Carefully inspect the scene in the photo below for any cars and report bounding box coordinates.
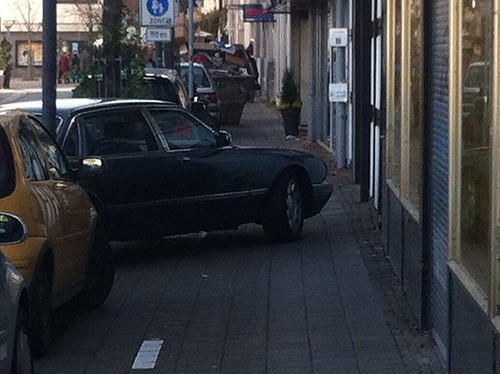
[0,214,36,372]
[0,110,117,348]
[165,62,223,125]
[1,99,333,241]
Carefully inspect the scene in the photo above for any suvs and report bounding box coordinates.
[71,67,208,122]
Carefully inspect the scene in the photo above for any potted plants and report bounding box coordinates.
[280,68,302,138]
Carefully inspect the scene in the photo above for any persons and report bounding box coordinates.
[56,51,81,84]
[277,67,303,140]
